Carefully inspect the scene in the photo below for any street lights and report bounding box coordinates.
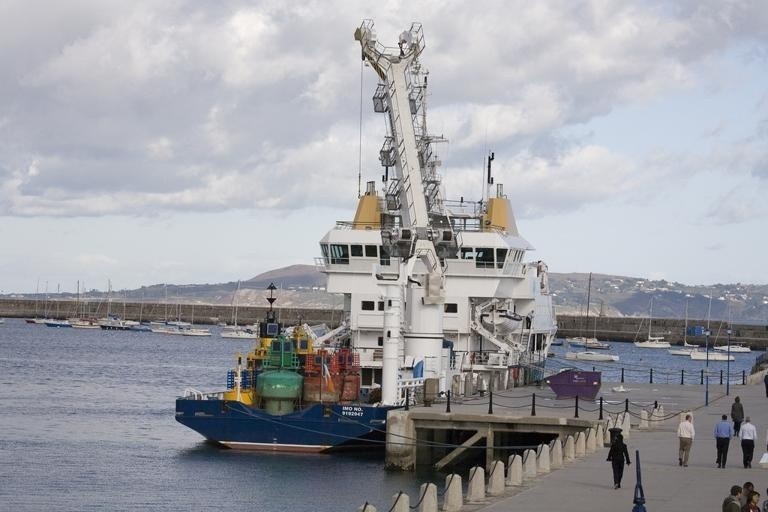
[703,327,711,407]
[726,328,733,397]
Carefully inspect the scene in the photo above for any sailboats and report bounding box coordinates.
[632,294,672,349]
[669,295,753,362]
[25,275,295,341]
[549,269,620,363]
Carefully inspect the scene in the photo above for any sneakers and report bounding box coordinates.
[732,432,740,437]
[742,461,753,469]
[717,462,727,469]
[678,458,689,467]
[613,483,621,489]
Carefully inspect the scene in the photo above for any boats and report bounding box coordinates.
[171,15,557,456]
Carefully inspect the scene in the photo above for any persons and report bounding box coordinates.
[739,416,757,468]
[713,415,732,468]
[723,482,768,511]
[606,434,631,489]
[677,415,695,466]
[764,372,768,396]
[731,396,745,436]
